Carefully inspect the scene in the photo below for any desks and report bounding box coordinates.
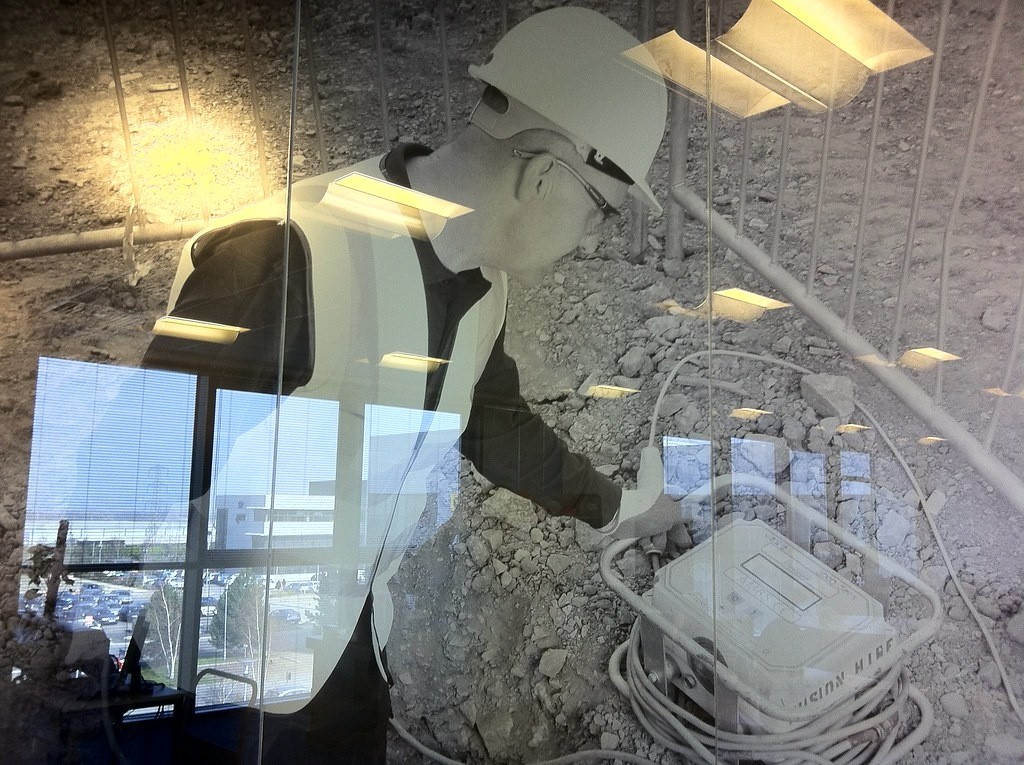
[19,680,184,765]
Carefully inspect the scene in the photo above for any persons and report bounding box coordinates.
[21,5,701,765]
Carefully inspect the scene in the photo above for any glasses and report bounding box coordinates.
[513,149,621,233]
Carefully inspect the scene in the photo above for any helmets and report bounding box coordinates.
[468,7,668,212]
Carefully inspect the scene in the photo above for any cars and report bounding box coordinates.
[18,563,321,672]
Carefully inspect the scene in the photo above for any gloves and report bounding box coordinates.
[596,443,698,553]
